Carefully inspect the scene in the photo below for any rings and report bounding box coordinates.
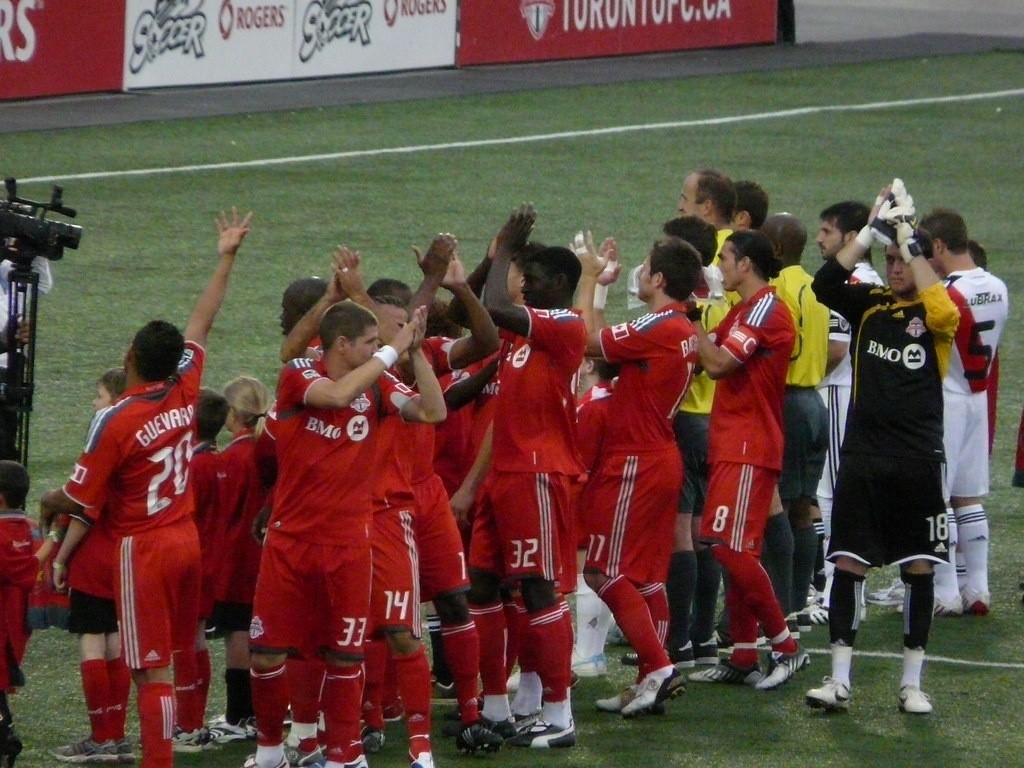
[341,267,348,272]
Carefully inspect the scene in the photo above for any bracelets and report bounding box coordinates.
[899,242,922,262]
[857,224,876,247]
[49,531,61,541]
[374,346,399,369]
[687,309,701,322]
[52,562,65,572]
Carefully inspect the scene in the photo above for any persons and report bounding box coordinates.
[1,167,1010,768]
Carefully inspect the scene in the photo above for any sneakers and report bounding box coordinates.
[242,698,435,768]
[866,577,905,606]
[456,719,503,757]
[688,657,762,686]
[52,735,118,763]
[621,665,688,719]
[596,684,637,712]
[171,727,224,753]
[606,583,868,666]
[504,720,575,749]
[960,584,991,616]
[806,676,850,712]
[896,595,964,617]
[755,639,811,690]
[115,736,137,765]
[210,713,257,743]
[898,684,932,713]
[0,721,22,768]
[429,644,608,728]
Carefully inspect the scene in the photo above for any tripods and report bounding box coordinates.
[6,263,40,468]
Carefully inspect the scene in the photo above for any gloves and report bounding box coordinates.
[884,194,923,264]
[870,178,914,246]
